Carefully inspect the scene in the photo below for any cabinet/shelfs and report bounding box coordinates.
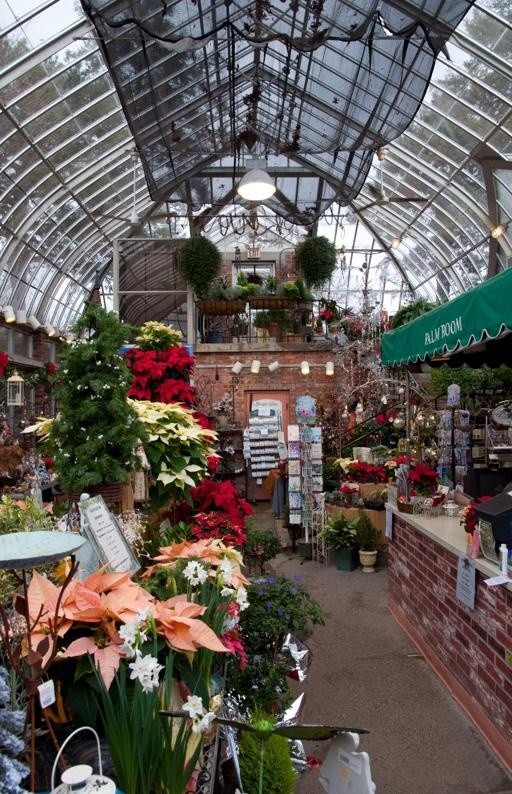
[507,549,512,580]
[498,543,508,576]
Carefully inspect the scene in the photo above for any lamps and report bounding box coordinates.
[2,305,334,381]
[491,219,512,238]
[376,146,390,204]
[237,152,276,201]
[127,154,141,225]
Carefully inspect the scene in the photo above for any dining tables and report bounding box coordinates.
[206,275,228,343]
[198,285,245,314]
[247,274,314,311]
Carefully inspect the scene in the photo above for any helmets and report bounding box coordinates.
[0,531,87,570]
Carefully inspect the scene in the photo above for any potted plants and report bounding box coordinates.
[247,274,314,311]
[206,275,228,343]
[198,285,245,314]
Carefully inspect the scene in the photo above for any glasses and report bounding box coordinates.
[127,154,141,225]
[237,152,276,201]
[376,146,390,204]
[491,219,512,238]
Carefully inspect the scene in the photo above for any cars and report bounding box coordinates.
[442,503,459,517]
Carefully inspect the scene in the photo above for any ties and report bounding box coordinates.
[475,481,512,553]
[464,467,511,497]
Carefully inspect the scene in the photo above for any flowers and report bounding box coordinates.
[0,303,439,793]
[460,495,493,534]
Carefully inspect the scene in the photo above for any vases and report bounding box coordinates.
[460,495,493,534]
